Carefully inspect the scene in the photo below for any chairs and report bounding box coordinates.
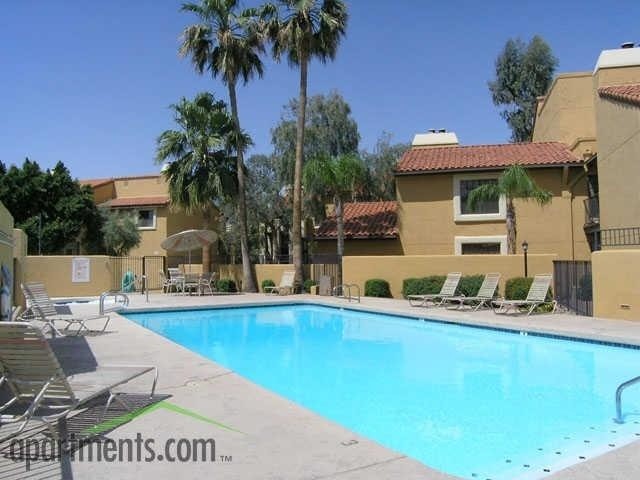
[264,270,296,296]
[17,281,110,337]
[441,272,502,312]
[491,273,557,316]
[0,322,159,452]
[159,268,216,297]
[407,272,462,306]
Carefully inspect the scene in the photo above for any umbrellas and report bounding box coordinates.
[160,228,218,294]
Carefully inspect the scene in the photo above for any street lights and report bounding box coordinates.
[522,240,528,278]
